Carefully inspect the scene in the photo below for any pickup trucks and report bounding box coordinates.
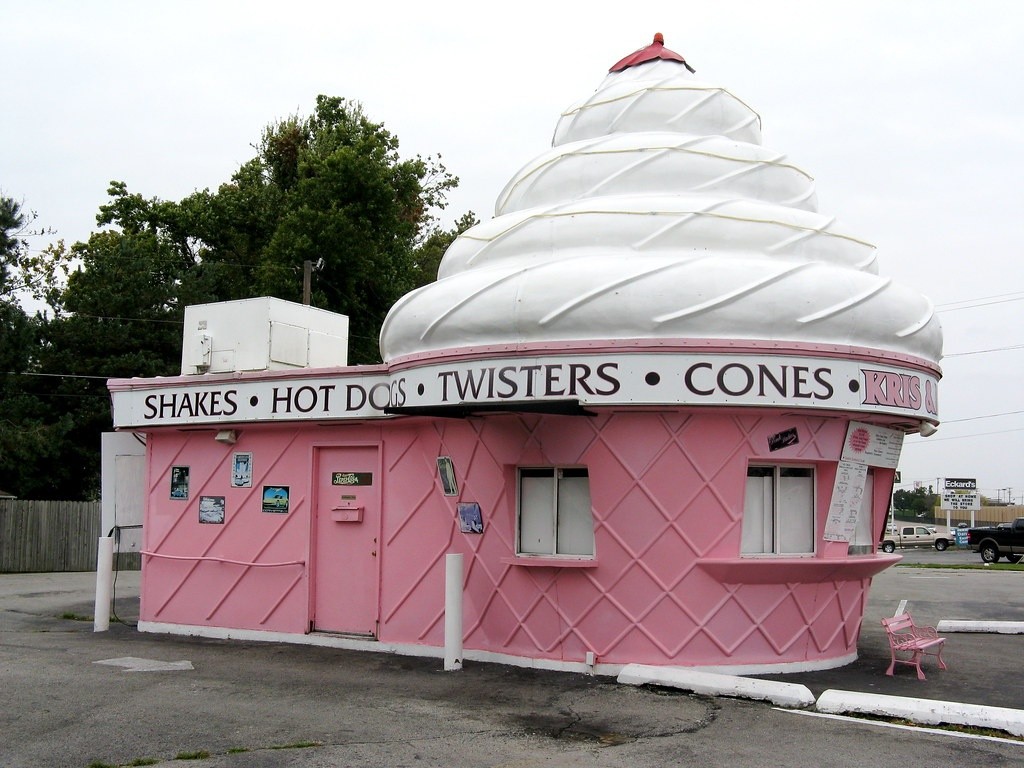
[877,525,955,553]
[966,517,1024,563]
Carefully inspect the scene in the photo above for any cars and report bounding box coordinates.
[886,521,897,532]
[949,527,957,537]
[958,522,967,528]
[924,525,937,534]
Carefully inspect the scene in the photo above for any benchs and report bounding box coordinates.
[880,611,946,680]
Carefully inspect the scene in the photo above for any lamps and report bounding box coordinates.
[919,420,938,437]
[215,429,236,444]
